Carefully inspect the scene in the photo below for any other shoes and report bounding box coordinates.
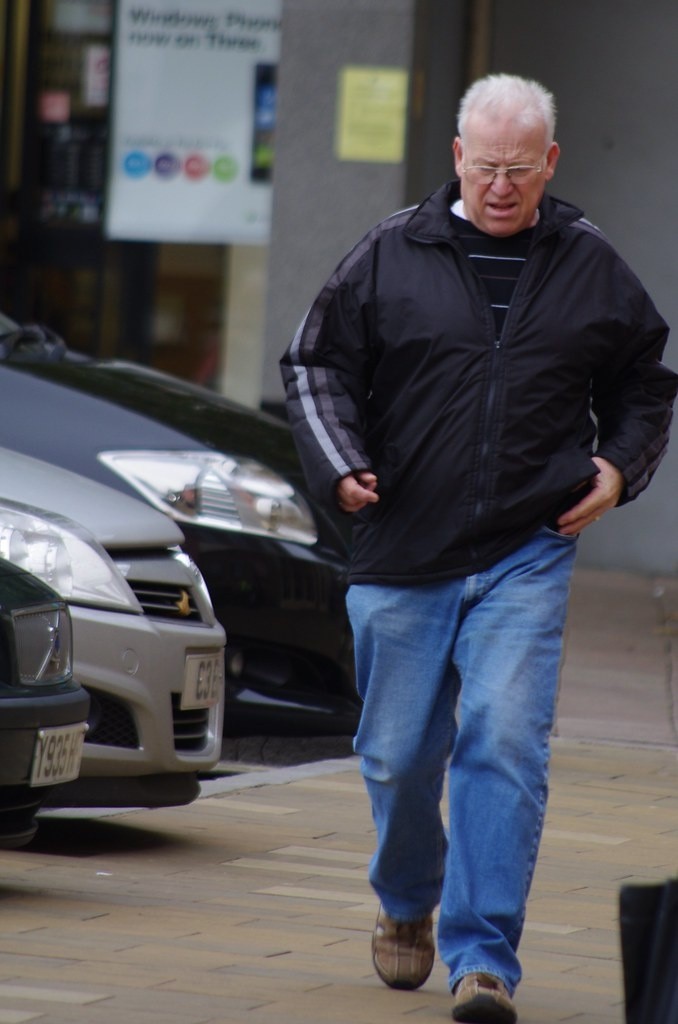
[371,903,435,990]
[452,974,518,1024]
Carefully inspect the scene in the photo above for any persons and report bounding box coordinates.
[280,73,678,1023]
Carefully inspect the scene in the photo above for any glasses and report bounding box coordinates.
[460,150,549,184]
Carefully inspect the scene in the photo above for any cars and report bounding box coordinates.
[0,445,226,810]
[0,313,365,730]
